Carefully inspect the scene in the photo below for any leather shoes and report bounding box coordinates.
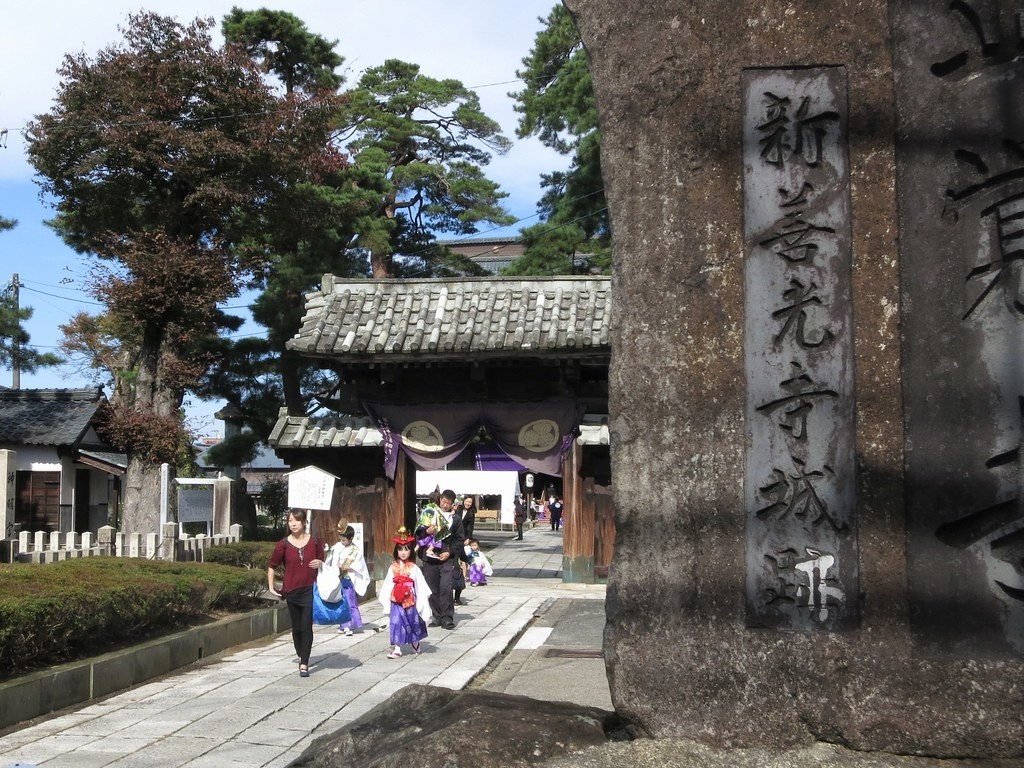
[441,616,455,629]
[429,621,442,627]
[299,656,309,677]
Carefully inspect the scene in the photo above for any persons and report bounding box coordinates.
[414,492,450,560]
[464,540,493,588]
[378,526,433,660]
[452,494,477,604]
[550,498,562,531]
[514,501,527,540]
[268,507,325,677]
[325,517,371,637]
[419,489,464,629]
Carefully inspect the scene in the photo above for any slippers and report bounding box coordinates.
[387,651,402,658]
[338,628,353,636]
[412,642,420,653]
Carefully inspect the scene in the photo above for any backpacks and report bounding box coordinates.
[523,513,527,522]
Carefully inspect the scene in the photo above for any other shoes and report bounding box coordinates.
[471,582,480,587]
[515,537,523,540]
[551,528,555,530]
[453,598,463,605]
[556,529,559,531]
